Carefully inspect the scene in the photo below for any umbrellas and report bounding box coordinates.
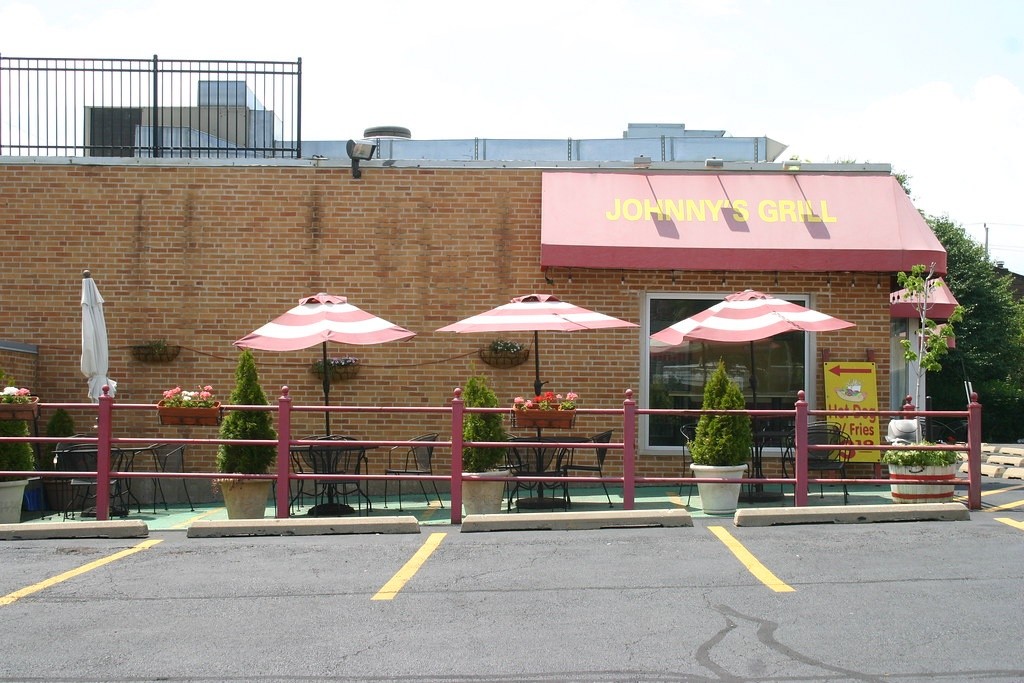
[80,271,116,401]
[233,292,417,502]
[649,290,857,493]
[436,294,642,499]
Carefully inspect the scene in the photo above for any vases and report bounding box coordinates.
[0,397,39,420]
[310,366,358,380]
[513,406,578,429]
[158,399,220,425]
[479,348,527,368]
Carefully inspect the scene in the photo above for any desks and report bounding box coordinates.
[739,431,788,501]
[288,445,376,514]
[48,443,166,517]
[507,435,587,510]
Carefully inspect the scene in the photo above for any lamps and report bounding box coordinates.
[634,154,801,171]
[345,139,376,178]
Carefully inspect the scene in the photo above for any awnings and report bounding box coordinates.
[539,173,947,276]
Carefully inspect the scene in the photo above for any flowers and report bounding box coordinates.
[515,392,577,410]
[158,385,216,408]
[0,387,30,404]
[316,357,357,365]
[489,340,521,353]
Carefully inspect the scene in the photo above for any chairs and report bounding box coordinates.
[57,431,614,519]
[678,422,853,504]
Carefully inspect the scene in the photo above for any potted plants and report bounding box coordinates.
[40,408,81,510]
[886,261,964,504]
[456,377,510,514]
[212,350,277,521]
[687,359,754,516]
[132,340,180,361]
[0,373,35,522]
